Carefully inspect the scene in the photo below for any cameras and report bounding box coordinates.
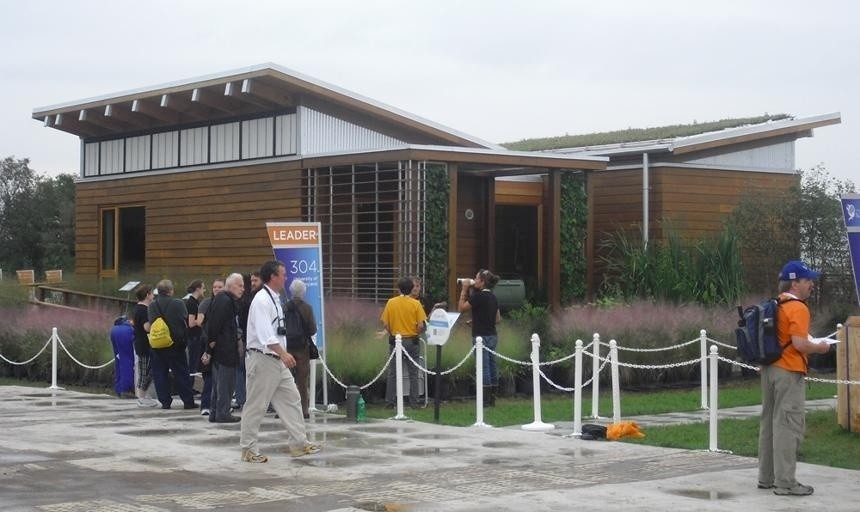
[278,327,287,335]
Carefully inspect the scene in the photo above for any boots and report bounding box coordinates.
[484,384,498,406]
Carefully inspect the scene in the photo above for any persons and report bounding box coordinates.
[375,276,432,402]
[239,259,323,463]
[380,276,425,407]
[458,269,501,405]
[112,264,321,425]
[755,260,832,494]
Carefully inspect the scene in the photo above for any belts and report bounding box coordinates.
[252,347,280,360]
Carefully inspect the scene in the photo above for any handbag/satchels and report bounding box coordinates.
[736,298,809,366]
[307,335,319,359]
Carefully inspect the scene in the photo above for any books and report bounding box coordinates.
[807,333,842,345]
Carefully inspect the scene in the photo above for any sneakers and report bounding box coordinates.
[118,389,200,409]
[757,480,776,488]
[291,442,322,459]
[201,407,240,423]
[772,482,814,496]
[241,447,267,464]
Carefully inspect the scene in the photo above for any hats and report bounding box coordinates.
[779,261,819,280]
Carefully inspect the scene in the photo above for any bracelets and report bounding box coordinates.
[459,293,467,299]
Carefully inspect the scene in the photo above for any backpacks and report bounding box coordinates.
[146,299,175,350]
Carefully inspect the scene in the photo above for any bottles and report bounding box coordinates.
[767,317,774,326]
[345,385,360,418]
[356,394,367,422]
[456,278,475,286]
